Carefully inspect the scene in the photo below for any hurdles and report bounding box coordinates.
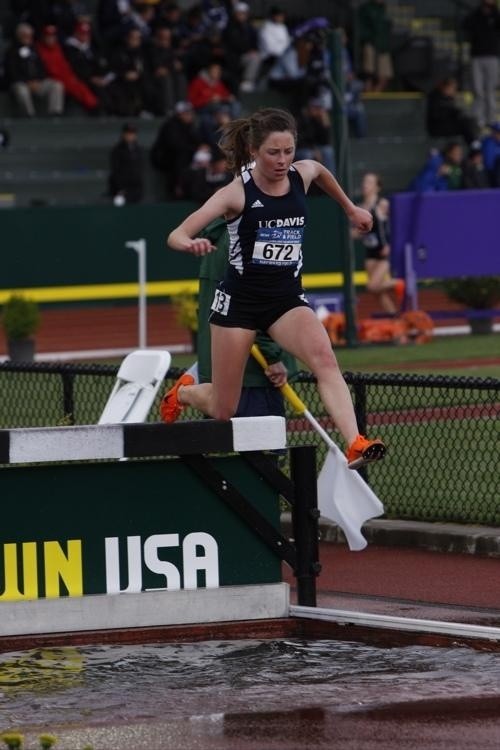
[2,416,324,639]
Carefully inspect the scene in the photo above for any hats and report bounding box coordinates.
[120,121,138,132]
[235,2,247,12]
[176,101,193,112]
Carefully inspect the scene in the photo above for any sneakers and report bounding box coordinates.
[345,436,383,469]
[157,375,195,422]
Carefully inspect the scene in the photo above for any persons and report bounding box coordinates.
[2,2,500,195]
[349,170,408,347]
[195,216,298,454]
[159,107,388,474]
